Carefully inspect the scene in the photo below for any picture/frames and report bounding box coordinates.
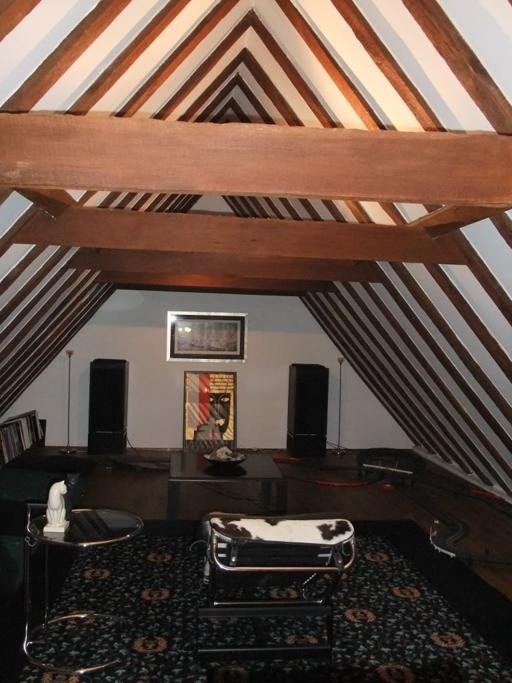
[164,309,248,364]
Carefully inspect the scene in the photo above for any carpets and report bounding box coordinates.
[7,516,512,683]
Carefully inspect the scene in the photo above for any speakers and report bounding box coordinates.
[87,358,129,455]
[286,363,330,458]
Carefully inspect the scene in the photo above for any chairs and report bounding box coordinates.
[181,508,360,680]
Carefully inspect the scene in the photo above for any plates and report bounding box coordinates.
[202,452,247,465]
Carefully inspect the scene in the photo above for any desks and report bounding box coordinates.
[21,506,147,677]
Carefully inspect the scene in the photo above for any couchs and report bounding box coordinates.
[0,410,95,534]
